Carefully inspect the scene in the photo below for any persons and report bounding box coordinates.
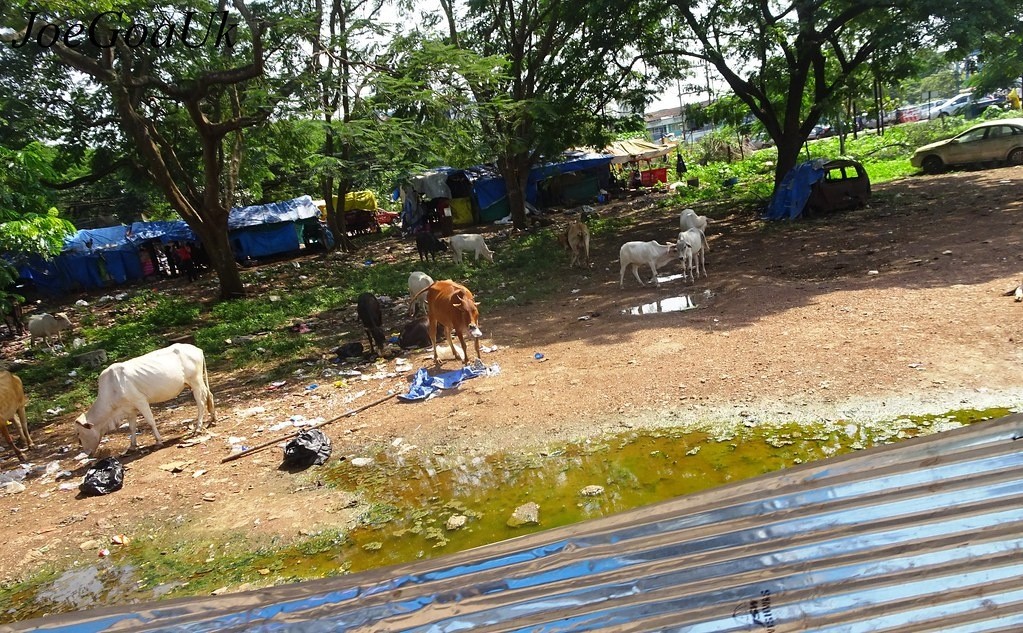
[140,240,210,282]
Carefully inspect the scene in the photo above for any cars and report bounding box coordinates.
[746,78,1023,151]
[910,118,1023,176]
[376,208,401,225]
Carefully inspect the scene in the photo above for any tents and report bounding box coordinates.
[1,225,143,295]
[224,193,325,260]
[130,220,197,247]
[415,137,680,224]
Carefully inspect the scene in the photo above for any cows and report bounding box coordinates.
[680,209,707,231]
[416,231,448,261]
[29,312,73,347]
[407,270,481,366]
[358,292,386,352]
[450,234,495,264]
[552,223,590,267]
[670,227,710,283]
[619,240,679,289]
[0,369,36,463]
[74,342,216,458]
